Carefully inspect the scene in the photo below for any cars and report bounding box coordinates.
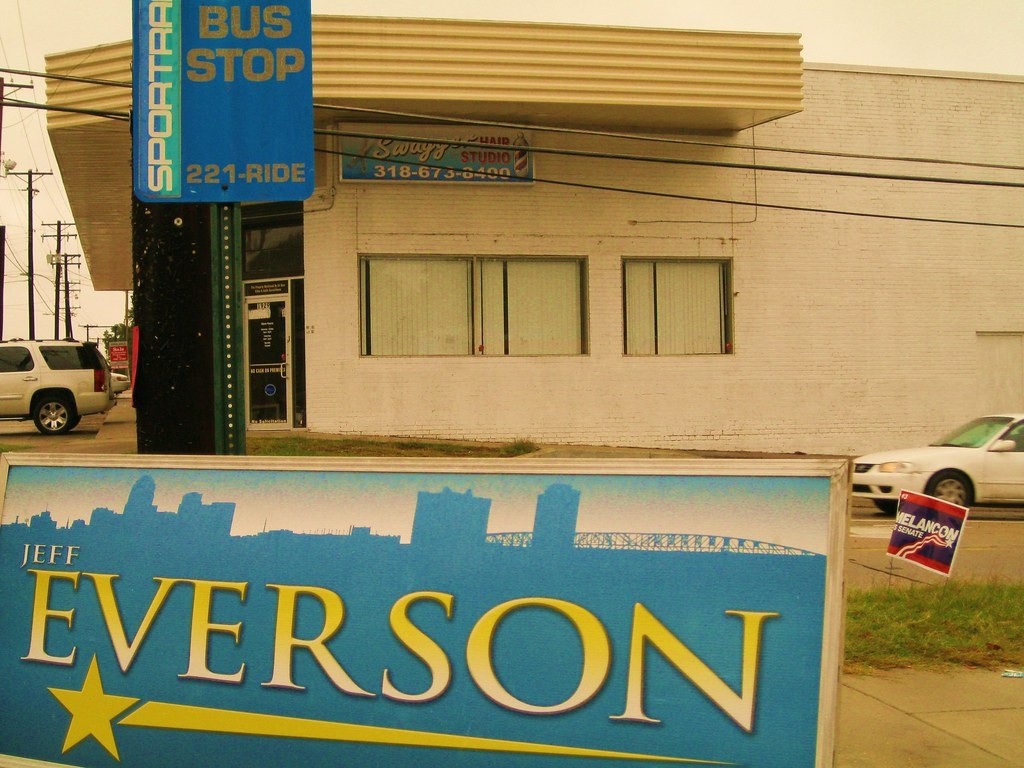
[848,414,1023,514]
[0,338,122,436]
[103,371,130,397]
